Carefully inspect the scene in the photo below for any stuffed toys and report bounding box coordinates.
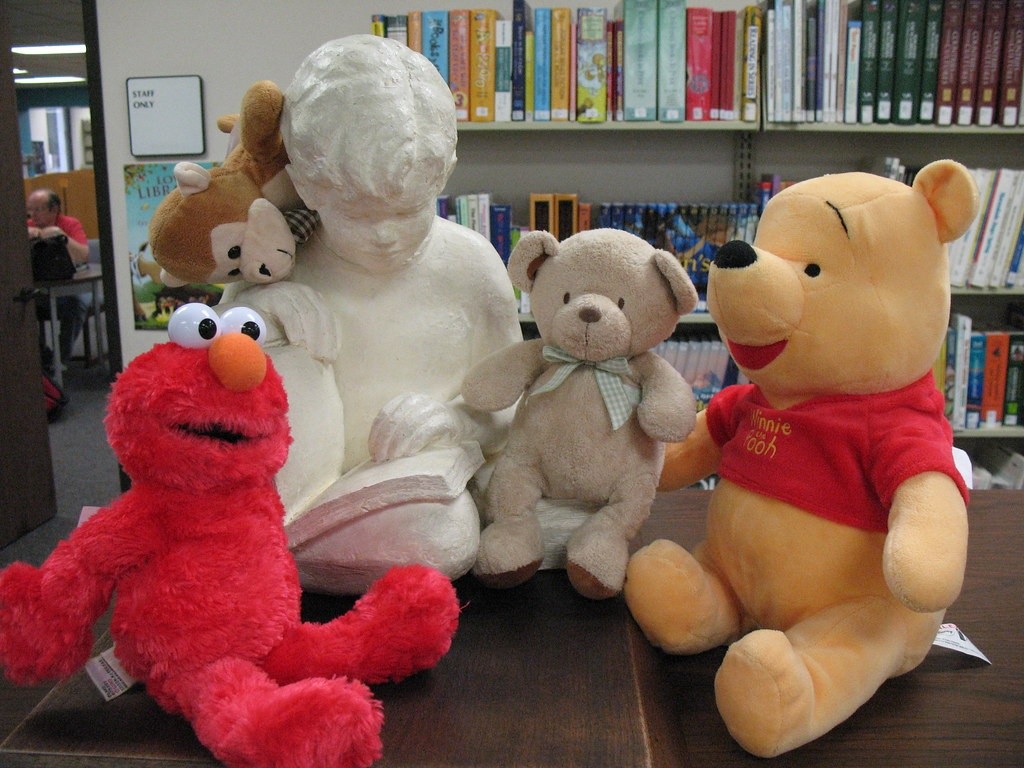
[622,158,980,757]
[461,227,698,601]
[1,303,460,768]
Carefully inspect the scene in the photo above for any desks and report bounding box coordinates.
[0,487,1024,768]
[36,262,103,390]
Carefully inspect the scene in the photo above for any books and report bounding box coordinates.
[882,157,1024,289]
[433,172,803,313]
[972,443,1024,491]
[648,329,752,412]
[933,302,1024,430]
[259,339,487,550]
[371,0,1024,126]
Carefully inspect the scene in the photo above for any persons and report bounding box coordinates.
[26,190,94,373]
[149,78,319,287]
[212,34,525,595]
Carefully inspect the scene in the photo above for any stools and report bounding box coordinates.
[38,298,106,368]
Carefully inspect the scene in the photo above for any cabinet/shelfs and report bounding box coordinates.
[760,121,1024,438]
[458,119,751,325]
